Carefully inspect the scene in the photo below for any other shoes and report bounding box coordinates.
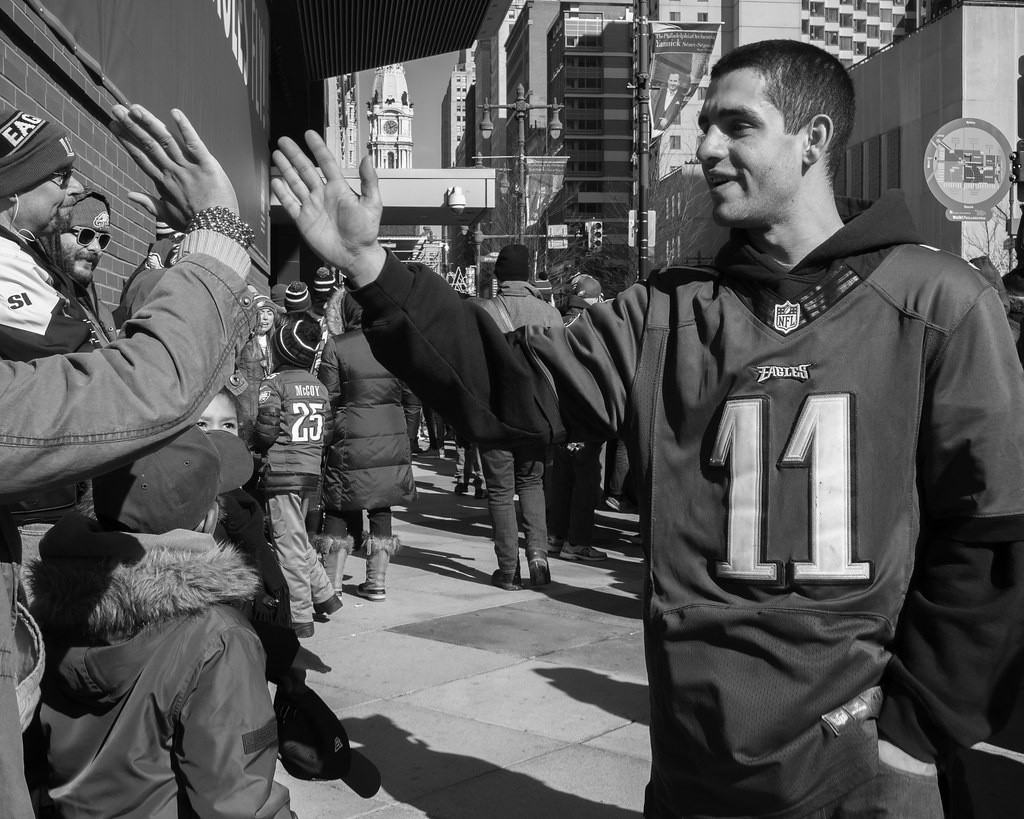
[474,490,488,498]
[314,595,343,615]
[356,582,386,602]
[439,450,445,458]
[292,623,314,638]
[418,446,440,459]
[455,484,468,493]
[606,496,633,512]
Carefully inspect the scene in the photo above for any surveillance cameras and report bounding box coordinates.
[447,194,467,215]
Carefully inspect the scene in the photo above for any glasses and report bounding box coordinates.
[71,226,111,250]
[52,169,73,187]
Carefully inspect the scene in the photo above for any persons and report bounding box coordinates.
[121,213,485,691]
[475,245,562,592]
[27,427,297,819]
[534,271,644,561]
[0,102,258,819]
[60,187,117,348]
[0,110,103,608]
[272,39,1024,819]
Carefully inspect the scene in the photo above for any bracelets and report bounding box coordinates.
[186,206,255,250]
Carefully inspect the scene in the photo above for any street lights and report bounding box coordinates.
[477,83,565,244]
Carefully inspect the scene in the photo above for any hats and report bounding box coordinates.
[271,284,288,307]
[313,267,336,294]
[69,196,110,234]
[575,276,601,298]
[272,319,322,367]
[0,109,76,198]
[493,245,530,281]
[284,281,311,311]
[272,675,382,800]
[91,423,255,535]
[534,272,553,295]
[253,293,277,317]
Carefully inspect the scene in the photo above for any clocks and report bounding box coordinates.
[384,121,398,135]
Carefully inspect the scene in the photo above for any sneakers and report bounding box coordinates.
[549,535,566,552]
[492,569,522,591]
[558,541,608,561]
[528,548,549,585]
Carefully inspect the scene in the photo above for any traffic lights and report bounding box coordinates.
[588,221,603,250]
